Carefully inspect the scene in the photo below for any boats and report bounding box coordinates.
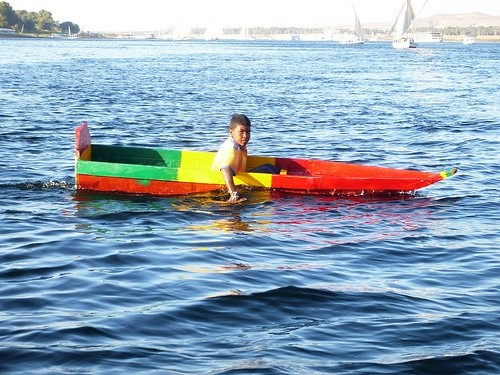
[74,120,458,197]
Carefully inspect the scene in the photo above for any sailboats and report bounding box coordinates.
[386,0,418,50]
[338,15,365,46]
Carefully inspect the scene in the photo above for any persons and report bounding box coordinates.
[212,114,286,206]
